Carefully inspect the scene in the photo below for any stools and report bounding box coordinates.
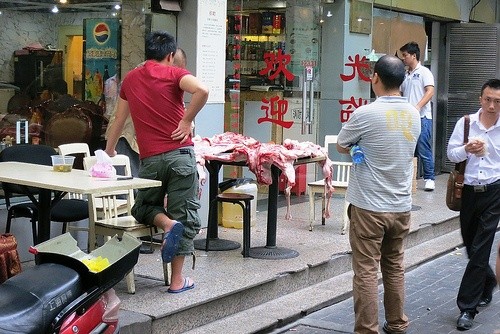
[205,193,255,257]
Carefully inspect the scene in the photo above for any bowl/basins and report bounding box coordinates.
[51,155,76,172]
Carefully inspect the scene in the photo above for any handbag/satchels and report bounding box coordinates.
[445,170,464,211]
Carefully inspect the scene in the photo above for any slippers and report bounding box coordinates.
[162,222,185,263]
[167,278,194,293]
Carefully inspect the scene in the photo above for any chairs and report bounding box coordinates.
[56,143,128,244]
[0,143,90,249]
[308,135,353,235]
[82,154,172,294]
[32,97,109,154]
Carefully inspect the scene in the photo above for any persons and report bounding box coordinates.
[98,99,106,111]
[447,79,500,330]
[399,41,435,189]
[49,79,81,113]
[39,90,51,106]
[337,55,422,334]
[106,29,209,292]
[104,47,186,198]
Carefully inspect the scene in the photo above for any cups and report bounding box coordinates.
[0,141,9,150]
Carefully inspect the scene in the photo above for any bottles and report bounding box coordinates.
[349,145,364,164]
[228,43,261,60]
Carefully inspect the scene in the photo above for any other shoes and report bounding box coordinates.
[457,312,473,330]
[383,321,389,332]
[423,179,435,191]
[478,281,497,307]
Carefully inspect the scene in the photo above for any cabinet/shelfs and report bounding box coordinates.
[225,7,286,93]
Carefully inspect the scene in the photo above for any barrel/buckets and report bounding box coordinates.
[222,183,257,229]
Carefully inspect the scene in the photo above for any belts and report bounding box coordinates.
[464,180,500,193]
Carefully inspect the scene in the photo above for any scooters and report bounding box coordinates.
[0,230,145,334]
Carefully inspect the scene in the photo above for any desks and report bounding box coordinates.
[0,161,161,266]
[193,154,327,260]
